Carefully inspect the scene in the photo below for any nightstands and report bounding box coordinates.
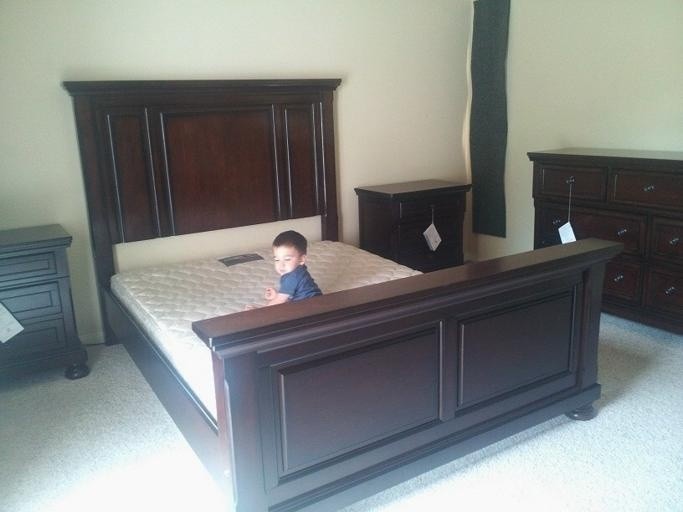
[354,178,471,274]
[1,222,89,380]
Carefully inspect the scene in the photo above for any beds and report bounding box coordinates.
[59,79,624,512]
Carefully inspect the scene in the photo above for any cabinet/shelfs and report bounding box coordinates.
[526,145,682,339]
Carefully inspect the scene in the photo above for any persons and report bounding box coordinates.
[242,230,323,312]
